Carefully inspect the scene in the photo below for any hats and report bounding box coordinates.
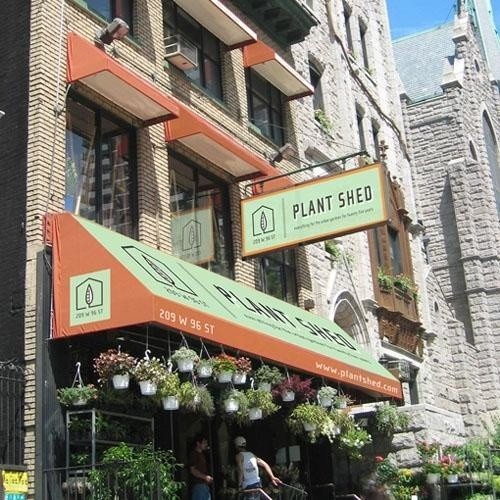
[234,436,246,447]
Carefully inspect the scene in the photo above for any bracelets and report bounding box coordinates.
[239,485,242,487]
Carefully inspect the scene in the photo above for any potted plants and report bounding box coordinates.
[246,351,404,460]
[92,348,250,412]
[420,461,466,485]
[56,383,97,407]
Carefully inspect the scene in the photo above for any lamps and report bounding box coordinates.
[270,142,297,165]
[94,16,130,47]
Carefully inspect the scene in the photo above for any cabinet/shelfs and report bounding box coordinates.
[67,412,155,498]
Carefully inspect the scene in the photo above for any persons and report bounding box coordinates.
[188,434,213,500]
[234,436,282,500]
